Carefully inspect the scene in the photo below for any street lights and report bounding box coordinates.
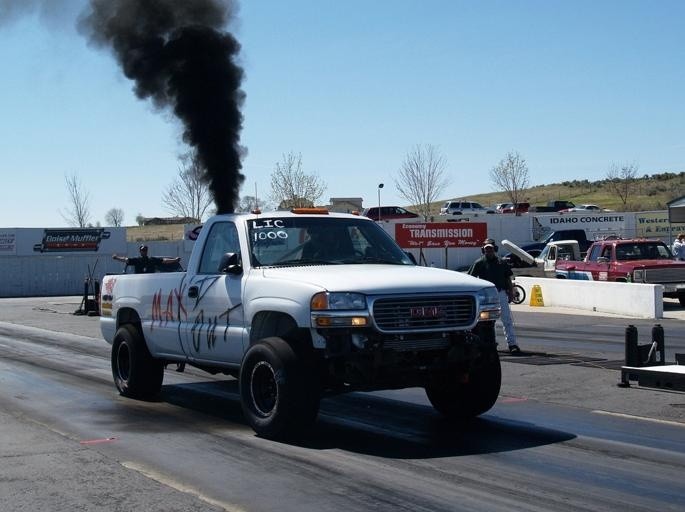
[377,182,388,221]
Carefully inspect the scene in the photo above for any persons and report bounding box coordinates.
[671,234,685,260]
[467,237,522,355]
[111,244,181,274]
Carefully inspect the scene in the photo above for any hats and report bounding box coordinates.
[140,245,147,250]
[483,239,496,248]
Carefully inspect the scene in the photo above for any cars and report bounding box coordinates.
[561,204,615,214]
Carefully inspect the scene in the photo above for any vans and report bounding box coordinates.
[498,203,531,212]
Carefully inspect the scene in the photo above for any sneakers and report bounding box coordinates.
[509,345,520,354]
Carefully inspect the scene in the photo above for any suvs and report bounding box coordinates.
[361,206,420,221]
[439,201,500,214]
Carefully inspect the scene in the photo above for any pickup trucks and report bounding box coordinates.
[527,201,575,212]
[506,228,685,307]
[97,205,506,442]
